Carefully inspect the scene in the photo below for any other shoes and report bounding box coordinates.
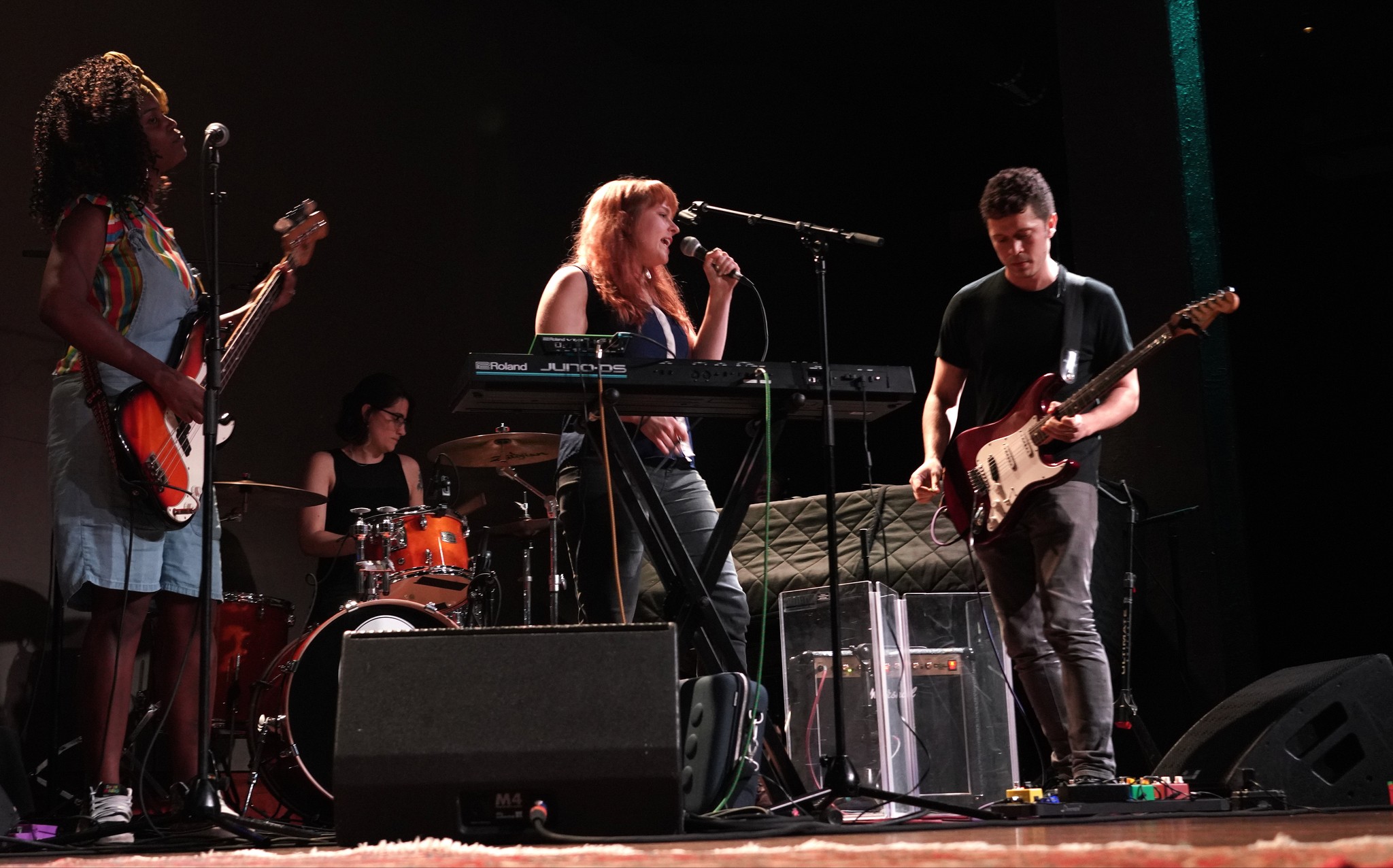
[1045,778,1106,797]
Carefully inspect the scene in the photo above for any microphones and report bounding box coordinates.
[680,236,756,288]
[204,121,229,147]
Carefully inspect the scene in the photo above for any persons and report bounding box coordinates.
[32,47,299,850]
[295,374,457,634]
[534,174,753,678]
[907,167,1141,786]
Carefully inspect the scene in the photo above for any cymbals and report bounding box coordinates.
[211,478,329,512]
[427,431,562,469]
[493,517,561,538]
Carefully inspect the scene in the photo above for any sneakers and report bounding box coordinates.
[171,775,243,840]
[85,783,137,845]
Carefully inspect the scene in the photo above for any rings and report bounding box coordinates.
[286,288,297,295]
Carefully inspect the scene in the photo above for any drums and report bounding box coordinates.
[214,590,296,741]
[252,598,467,828]
[358,505,475,616]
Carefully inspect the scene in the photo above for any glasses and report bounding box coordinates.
[372,405,409,428]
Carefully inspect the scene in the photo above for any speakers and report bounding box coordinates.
[1146,653,1393,809]
[328,623,682,844]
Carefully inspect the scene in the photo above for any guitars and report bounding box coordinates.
[101,199,328,533]
[940,288,1242,550]
